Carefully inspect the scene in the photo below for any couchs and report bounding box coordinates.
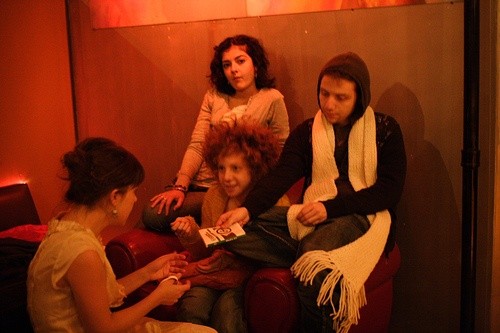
[102,174,401,333]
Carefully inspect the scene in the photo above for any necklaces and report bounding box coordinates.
[77,203,105,251]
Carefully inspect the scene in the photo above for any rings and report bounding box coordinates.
[163,197,167,202]
[161,194,165,198]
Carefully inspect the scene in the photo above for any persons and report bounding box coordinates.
[26,135,219,332]
[170,120,292,332]
[142,33,290,232]
[214,51,407,332]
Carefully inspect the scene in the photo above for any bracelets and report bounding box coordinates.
[172,187,186,192]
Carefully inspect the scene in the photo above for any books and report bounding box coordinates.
[198,221,247,249]
